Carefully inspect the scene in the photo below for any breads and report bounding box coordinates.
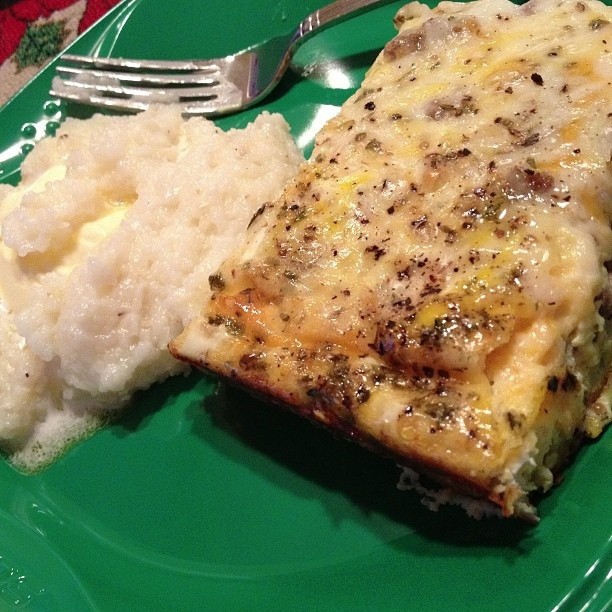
[170,4,612,530]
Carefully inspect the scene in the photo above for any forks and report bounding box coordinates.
[52,1,402,116]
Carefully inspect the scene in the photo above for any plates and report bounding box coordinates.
[0,1,611,607]
